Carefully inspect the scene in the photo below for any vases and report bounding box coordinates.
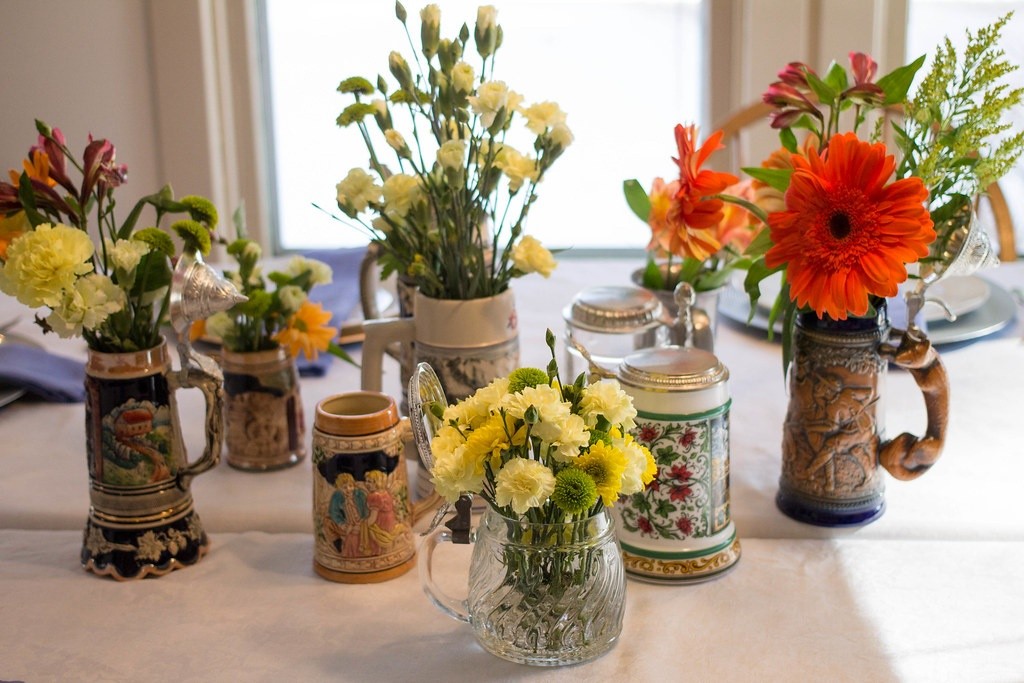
[78,331,225,581]
[773,302,951,529]
[202,346,308,474]
[630,263,721,339]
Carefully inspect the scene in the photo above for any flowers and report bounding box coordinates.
[623,122,756,293]
[178,199,388,377]
[311,0,575,300]
[714,9,1024,396]
[425,327,658,524]
[0,117,219,354]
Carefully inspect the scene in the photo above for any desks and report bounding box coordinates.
[0,256,1023,540]
[0,527,1024,683]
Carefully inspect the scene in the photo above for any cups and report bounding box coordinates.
[565,335,742,585]
[297,391,447,583]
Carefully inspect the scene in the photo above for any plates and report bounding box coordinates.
[712,270,1017,348]
